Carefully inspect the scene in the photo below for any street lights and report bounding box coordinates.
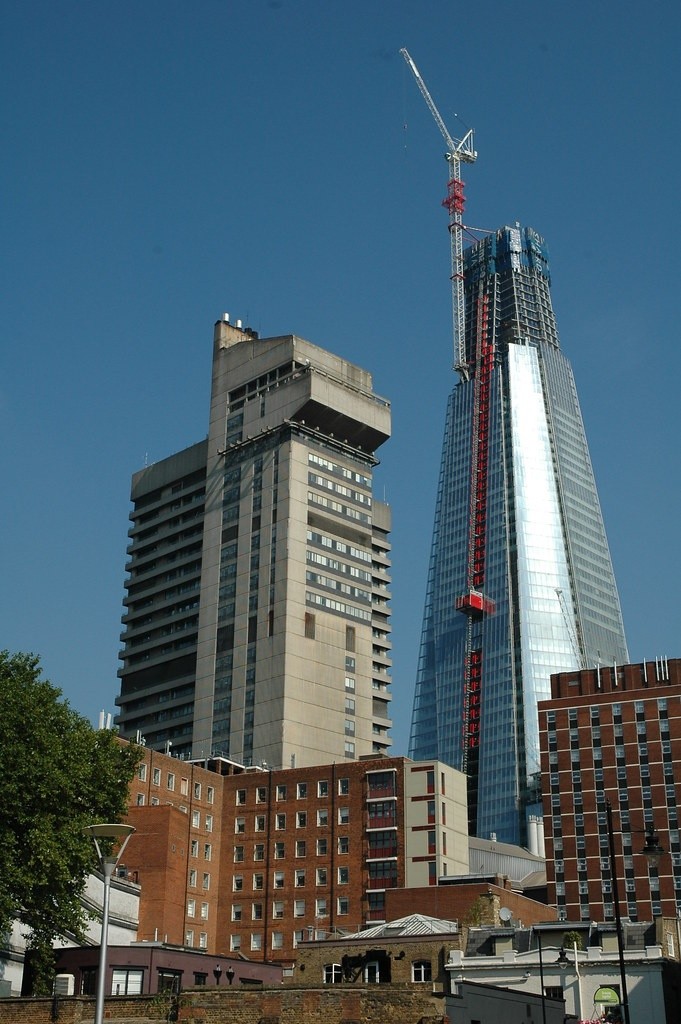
[81,821,137,1024]
[225,966,234,985]
[602,800,665,1024]
[535,929,568,1023]
[213,964,222,985]
